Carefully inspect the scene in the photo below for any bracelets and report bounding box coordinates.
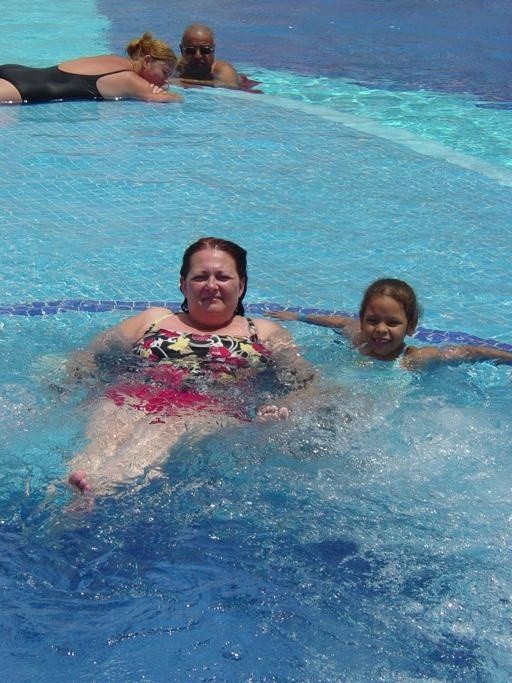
[297,311,308,323]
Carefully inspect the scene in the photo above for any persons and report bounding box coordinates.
[0,29,180,104]
[256,275,511,459]
[163,23,264,95]
[45,234,319,514]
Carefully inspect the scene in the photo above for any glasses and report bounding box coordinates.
[183,47,214,54]
